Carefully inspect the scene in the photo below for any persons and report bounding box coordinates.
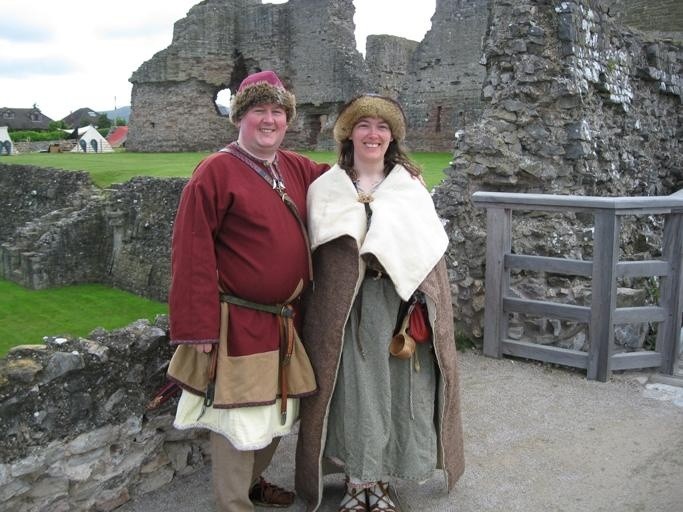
[143,64,430,512]
[293,91,466,512]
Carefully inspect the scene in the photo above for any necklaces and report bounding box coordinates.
[353,176,387,205]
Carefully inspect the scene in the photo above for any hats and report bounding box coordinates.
[228,70,296,126]
[333,93,405,145]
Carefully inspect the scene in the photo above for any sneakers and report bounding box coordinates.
[338,487,366,512]
[248,477,294,509]
[367,482,395,512]
